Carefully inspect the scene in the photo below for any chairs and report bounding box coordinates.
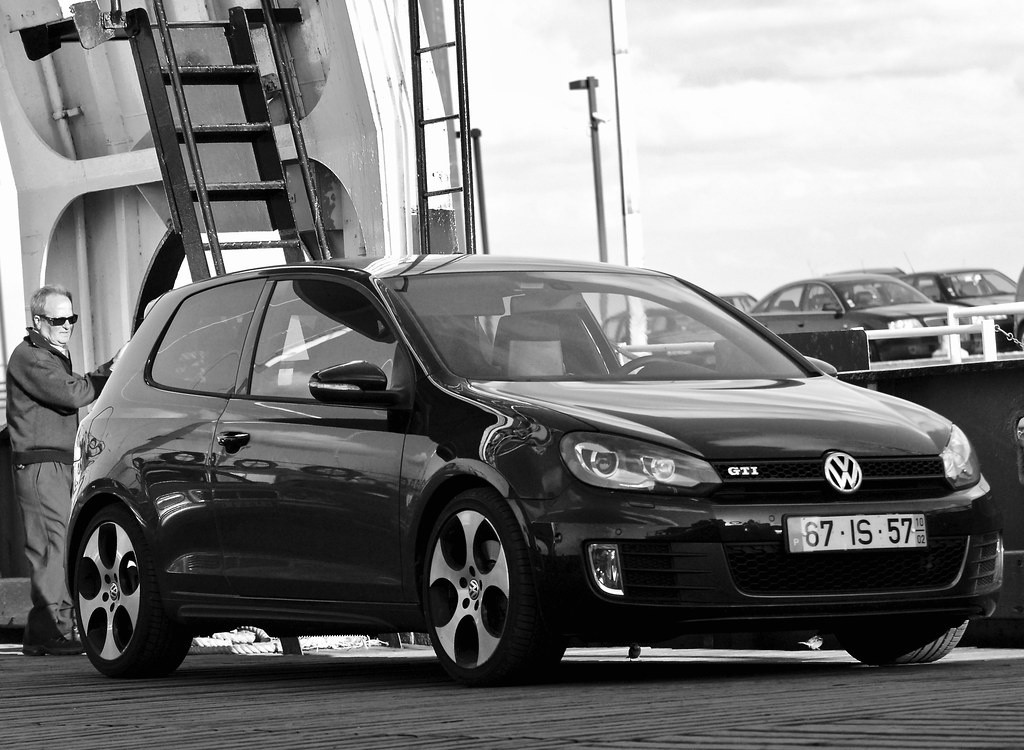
[779,300,797,309]
[492,314,565,377]
[960,283,978,295]
[854,291,874,306]
[812,294,833,312]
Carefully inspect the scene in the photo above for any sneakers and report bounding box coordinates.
[23,625,83,656]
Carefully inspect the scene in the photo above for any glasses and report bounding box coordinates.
[39,314,78,325]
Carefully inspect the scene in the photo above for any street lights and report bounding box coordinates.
[567,77,609,265]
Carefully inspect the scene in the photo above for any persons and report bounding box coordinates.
[6,284,129,656]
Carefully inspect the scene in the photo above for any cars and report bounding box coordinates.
[603,264,1024,373]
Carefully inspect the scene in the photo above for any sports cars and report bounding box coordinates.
[60,250,1005,688]
